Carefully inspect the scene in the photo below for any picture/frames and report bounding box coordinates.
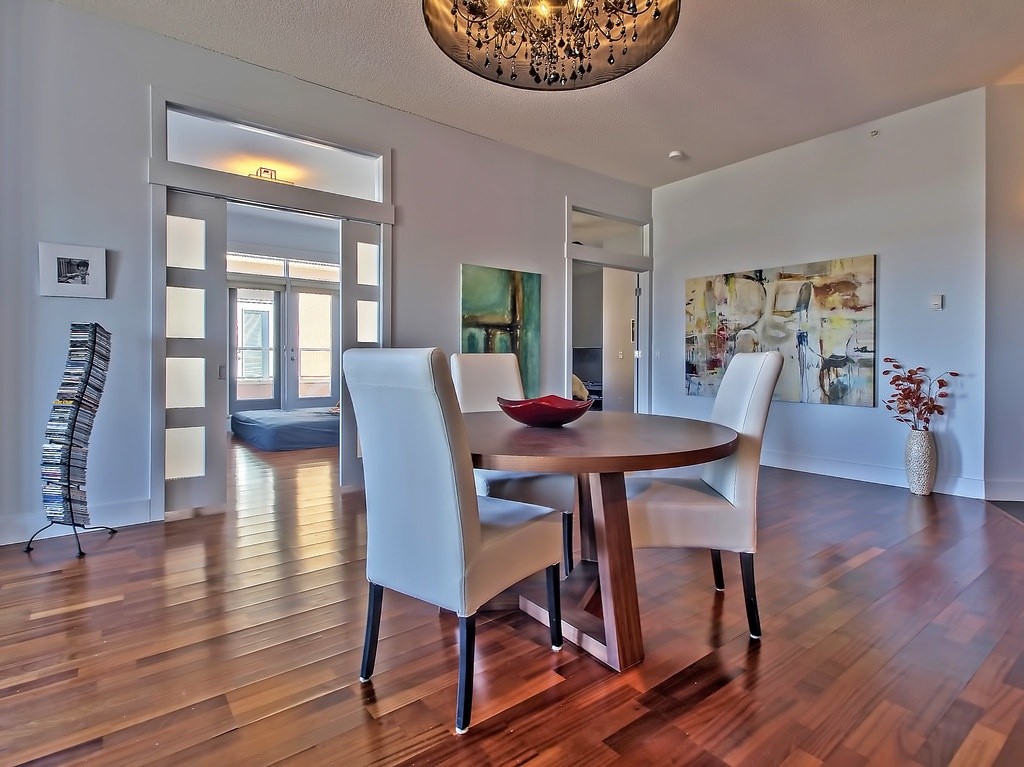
[38,241,106,299]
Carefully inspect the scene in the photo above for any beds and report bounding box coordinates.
[231,407,338,453]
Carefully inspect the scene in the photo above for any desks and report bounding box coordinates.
[439,411,739,673]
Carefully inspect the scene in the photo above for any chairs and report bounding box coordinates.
[450,352,577,577]
[343,347,563,735]
[624,351,784,639]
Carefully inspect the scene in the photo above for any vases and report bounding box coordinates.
[904,429,938,496]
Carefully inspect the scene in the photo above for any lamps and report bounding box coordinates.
[422,0,681,92]
[248,167,294,186]
